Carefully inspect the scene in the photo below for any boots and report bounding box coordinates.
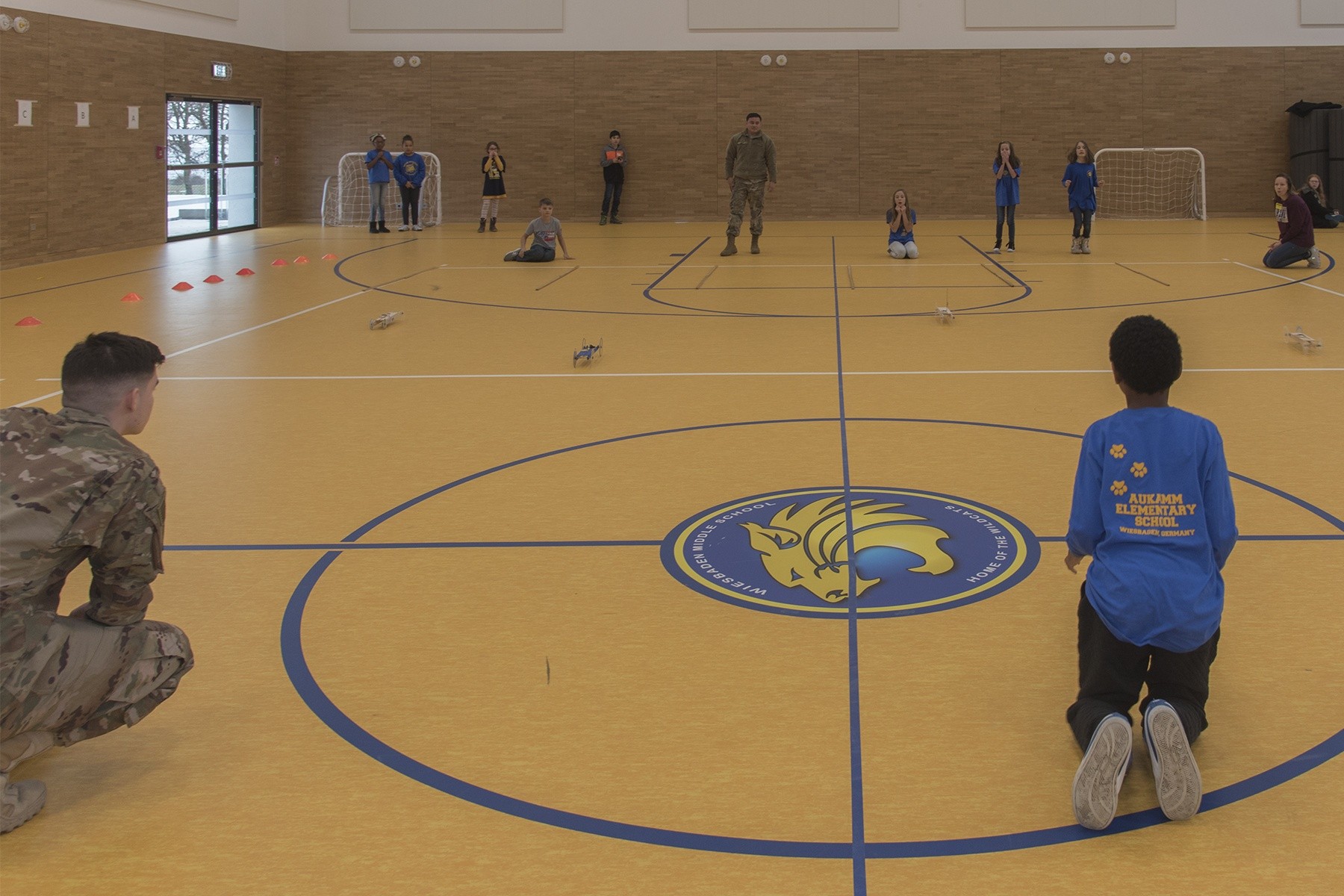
[1081,237,1091,253]
[369,222,379,233]
[478,217,486,233]
[379,221,390,233]
[1070,235,1082,253]
[751,234,760,253]
[490,217,497,232]
[720,235,738,256]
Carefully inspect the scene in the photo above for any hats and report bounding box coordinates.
[369,133,387,142]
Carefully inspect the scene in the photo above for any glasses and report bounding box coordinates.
[374,141,385,144]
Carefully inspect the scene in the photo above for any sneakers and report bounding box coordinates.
[399,224,410,231]
[609,215,622,224]
[1144,697,1204,822]
[504,248,522,262]
[0,771,47,836]
[1074,707,1135,830]
[411,224,422,231]
[994,239,1003,251]
[1006,242,1016,252]
[1306,248,1320,268]
[599,216,608,224]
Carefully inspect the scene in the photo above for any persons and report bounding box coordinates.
[992,140,1021,252]
[477,141,507,233]
[719,112,779,257]
[1060,314,1240,831]
[1060,140,1105,254]
[503,197,576,263]
[1262,172,1322,269]
[0,328,196,836]
[1296,174,1340,229]
[392,134,426,231]
[886,188,919,259]
[598,129,628,225]
[363,133,394,234]
